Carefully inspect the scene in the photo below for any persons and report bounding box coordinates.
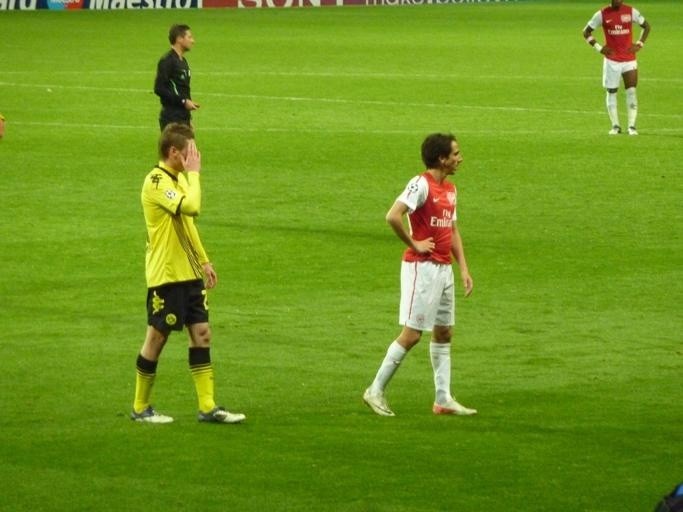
[582,0,650,136]
[361,130,478,417]
[152,22,200,132]
[129,120,247,423]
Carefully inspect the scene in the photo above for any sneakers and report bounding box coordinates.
[198,407,246,423]
[131,405,173,424]
[362,387,395,417]
[433,400,477,416]
[609,127,638,135]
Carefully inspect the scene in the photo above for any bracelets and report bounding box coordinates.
[635,40,643,48]
[585,35,594,43]
[592,42,603,53]
